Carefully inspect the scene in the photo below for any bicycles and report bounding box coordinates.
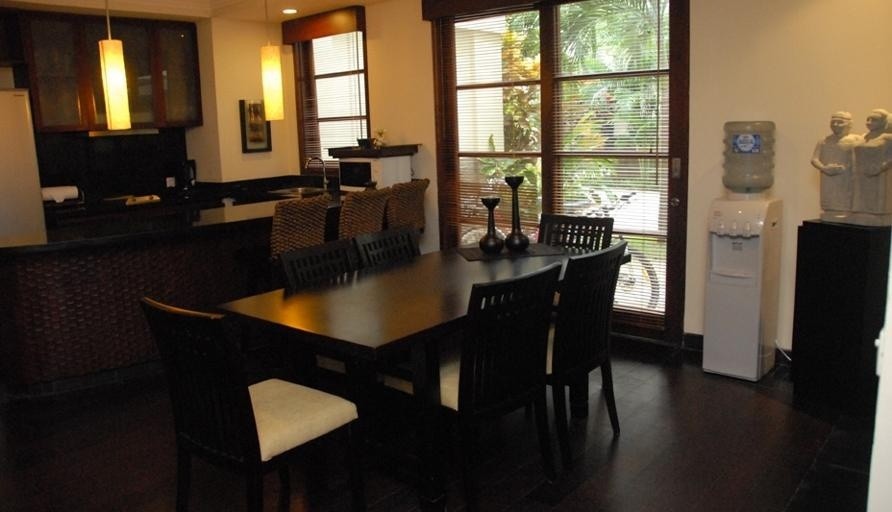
[461,184,659,309]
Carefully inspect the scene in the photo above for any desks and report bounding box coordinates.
[216,231,630,512]
[791,214,891,437]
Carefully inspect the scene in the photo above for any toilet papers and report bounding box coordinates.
[41,186,79,203]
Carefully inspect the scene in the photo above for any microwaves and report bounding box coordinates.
[339,155,412,192]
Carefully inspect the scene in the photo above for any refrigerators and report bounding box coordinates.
[1,89,49,250]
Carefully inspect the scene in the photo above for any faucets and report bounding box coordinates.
[304,156,329,189]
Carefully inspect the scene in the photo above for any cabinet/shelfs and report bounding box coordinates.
[16,13,203,133]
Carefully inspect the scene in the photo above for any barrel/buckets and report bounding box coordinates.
[721,120,776,194]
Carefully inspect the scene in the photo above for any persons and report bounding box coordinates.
[810,110,860,213]
[854,108,892,216]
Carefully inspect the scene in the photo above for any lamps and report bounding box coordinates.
[257,0,286,121]
[98,0,132,131]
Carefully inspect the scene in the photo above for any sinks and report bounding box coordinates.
[267,186,323,198]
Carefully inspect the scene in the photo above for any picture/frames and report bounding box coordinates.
[239,98,271,152]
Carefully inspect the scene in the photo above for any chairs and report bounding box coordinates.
[268,177,430,258]
[352,222,421,269]
[537,214,614,311]
[276,237,359,468]
[138,293,367,512]
[524,240,627,443]
[374,260,562,512]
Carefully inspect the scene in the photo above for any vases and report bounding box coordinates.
[480,197,505,255]
[505,176,529,253]
